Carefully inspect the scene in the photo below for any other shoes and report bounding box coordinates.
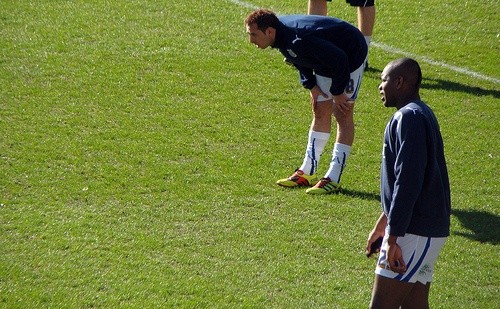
[284,57,293,65]
[365,60,368,70]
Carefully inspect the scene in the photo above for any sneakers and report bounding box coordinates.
[306,177,341,194]
[277,167,320,186]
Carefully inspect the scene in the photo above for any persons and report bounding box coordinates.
[366,58,451,309]
[244,8,368,194]
[308,0,375,71]
